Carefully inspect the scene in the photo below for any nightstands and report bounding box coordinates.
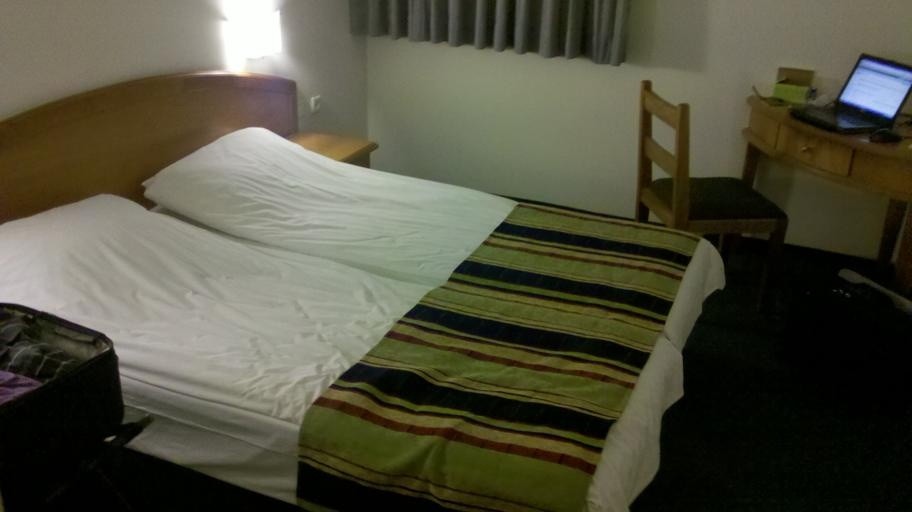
[284,129,380,169]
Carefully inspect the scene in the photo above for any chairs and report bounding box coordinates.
[634,78,789,247]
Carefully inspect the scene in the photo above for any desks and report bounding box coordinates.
[740,92,910,264]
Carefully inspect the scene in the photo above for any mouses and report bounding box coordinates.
[871,126,902,143]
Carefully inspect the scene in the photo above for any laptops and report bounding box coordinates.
[790,51,912,136]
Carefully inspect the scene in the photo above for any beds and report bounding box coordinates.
[0,69,712,512]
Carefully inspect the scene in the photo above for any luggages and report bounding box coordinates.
[0,301,126,480]
